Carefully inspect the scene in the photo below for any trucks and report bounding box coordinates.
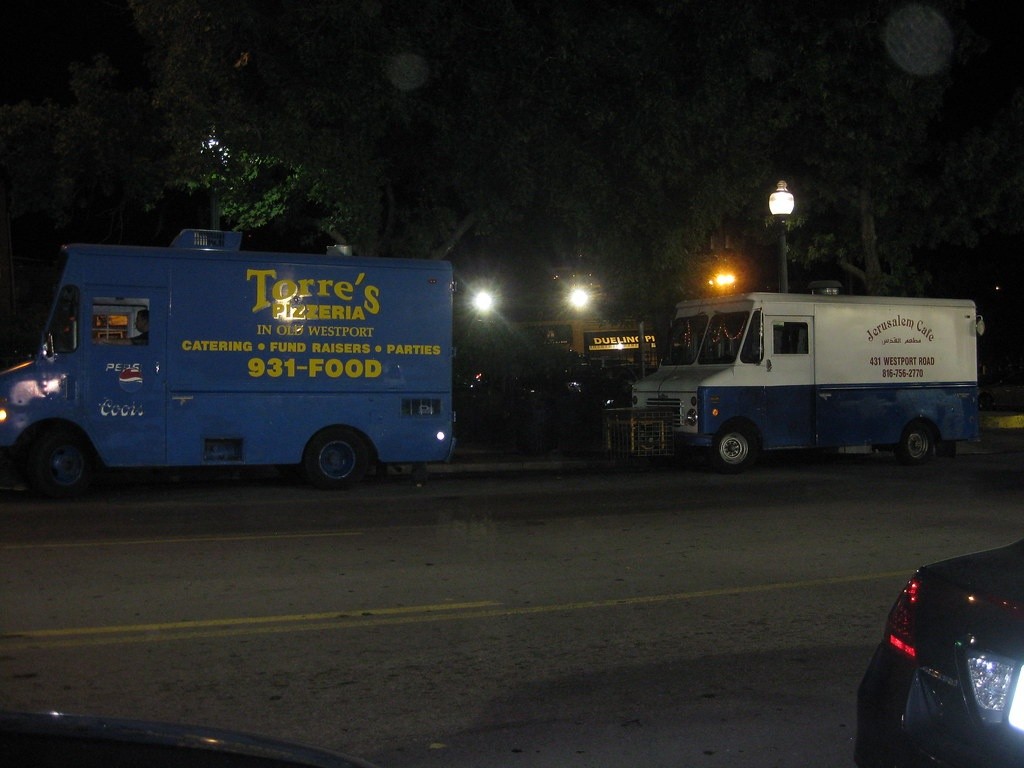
[453,318,668,456]
[632,281,984,473]
[0,230,455,498]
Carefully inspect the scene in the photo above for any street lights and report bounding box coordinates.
[769,180,795,294]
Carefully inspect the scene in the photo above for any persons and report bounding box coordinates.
[97,310,149,345]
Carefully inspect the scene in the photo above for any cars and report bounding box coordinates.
[854,535,1024,768]
[977,370,1024,412]
